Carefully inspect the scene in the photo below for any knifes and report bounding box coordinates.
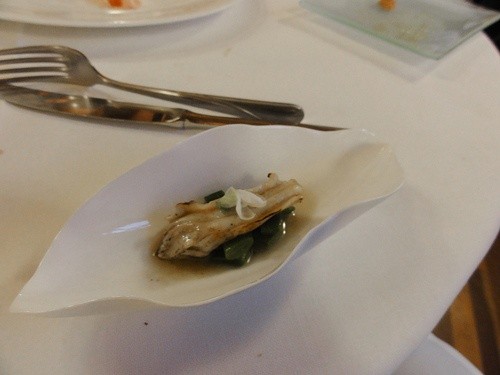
[2,82,351,134]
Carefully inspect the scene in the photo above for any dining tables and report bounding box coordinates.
[399,1,500,373]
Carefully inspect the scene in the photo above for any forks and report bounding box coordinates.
[0,46,305,123]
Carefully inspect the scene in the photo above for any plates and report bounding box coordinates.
[6,122,405,316]
[293,0,500,60]
[1,0,237,30]
